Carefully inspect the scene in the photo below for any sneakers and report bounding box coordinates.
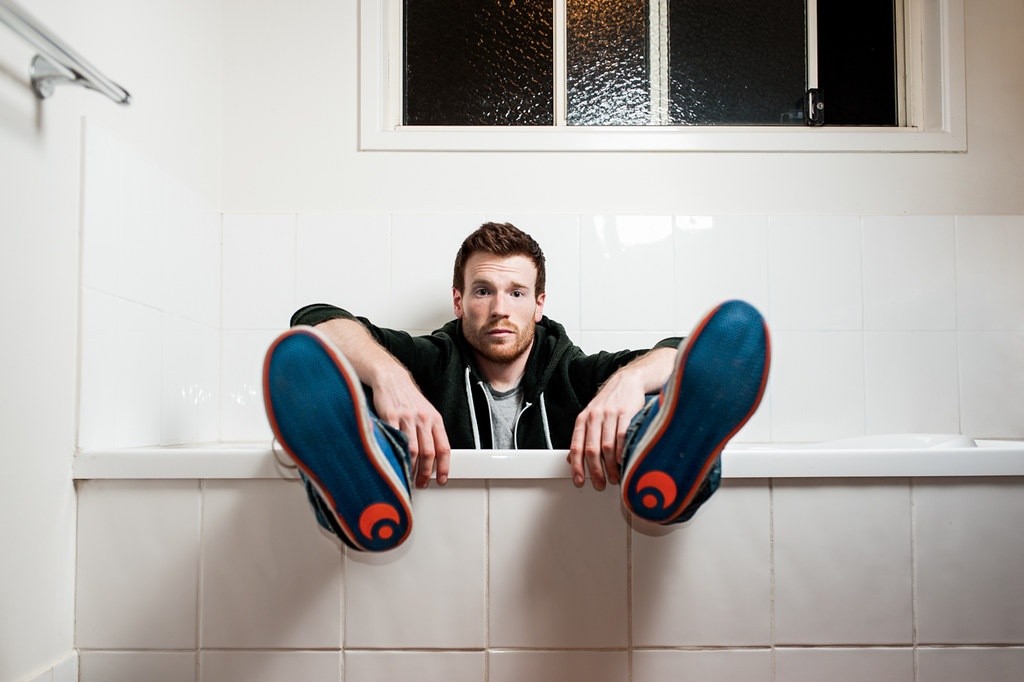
[619,299,771,525]
[262,323,412,554]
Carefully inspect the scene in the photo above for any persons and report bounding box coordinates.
[261,222,771,553]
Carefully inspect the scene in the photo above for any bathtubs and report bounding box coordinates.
[75,432,1024,681]
